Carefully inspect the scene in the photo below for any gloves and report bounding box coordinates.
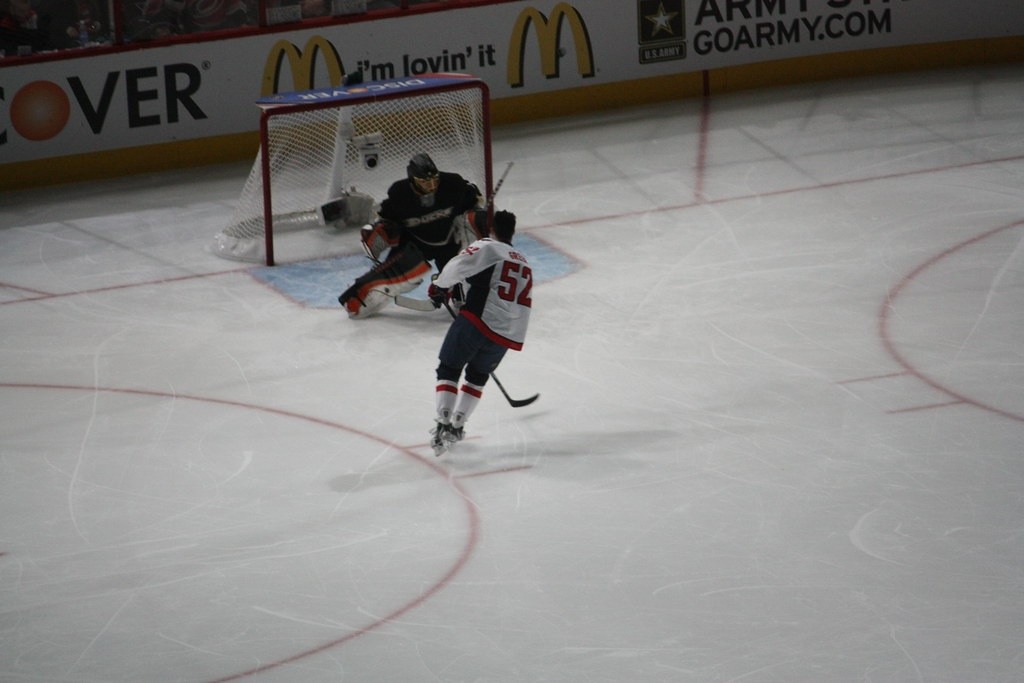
[428,285,451,306]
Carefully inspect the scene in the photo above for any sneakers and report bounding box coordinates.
[429,423,444,455]
[446,423,466,452]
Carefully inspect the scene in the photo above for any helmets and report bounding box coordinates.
[407,153,441,195]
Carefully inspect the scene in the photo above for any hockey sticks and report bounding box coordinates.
[393,160,515,312]
[442,297,540,408]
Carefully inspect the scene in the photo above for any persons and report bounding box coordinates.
[0,0,402,57]
[428,209,534,455]
[339,150,487,320]
